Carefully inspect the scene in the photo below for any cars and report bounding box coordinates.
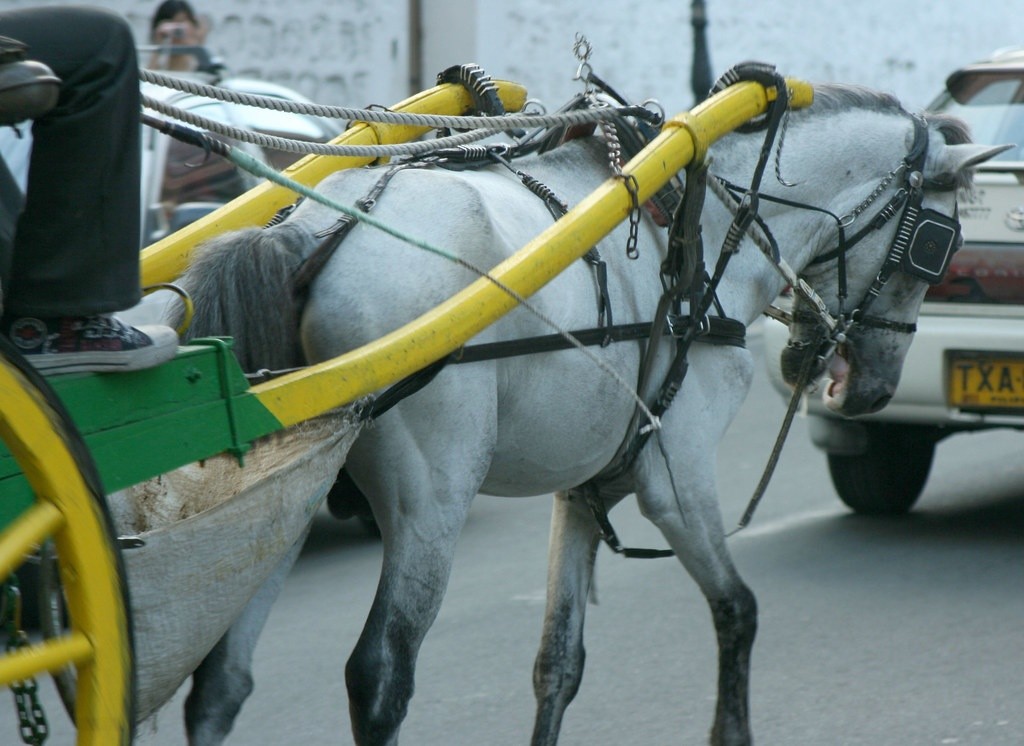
[0,65,348,324]
[806,40,1024,518]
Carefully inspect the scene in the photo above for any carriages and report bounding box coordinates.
[0,40,1020,746]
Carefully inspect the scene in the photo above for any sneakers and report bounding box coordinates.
[1,309,179,376]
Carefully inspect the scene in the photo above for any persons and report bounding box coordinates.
[148,1,246,204]
[0,6,179,374]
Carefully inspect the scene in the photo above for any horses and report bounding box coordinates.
[186,87,1016,746]
